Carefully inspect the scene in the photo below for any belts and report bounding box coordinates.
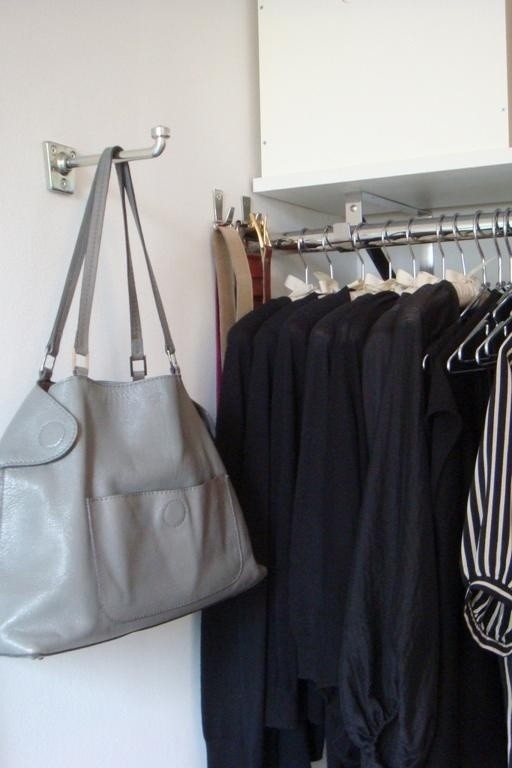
[251,212,272,303]
[213,225,253,373]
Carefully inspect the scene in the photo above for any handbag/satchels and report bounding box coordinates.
[0,146,267,654]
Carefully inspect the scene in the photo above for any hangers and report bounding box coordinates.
[283,209,512,374]
